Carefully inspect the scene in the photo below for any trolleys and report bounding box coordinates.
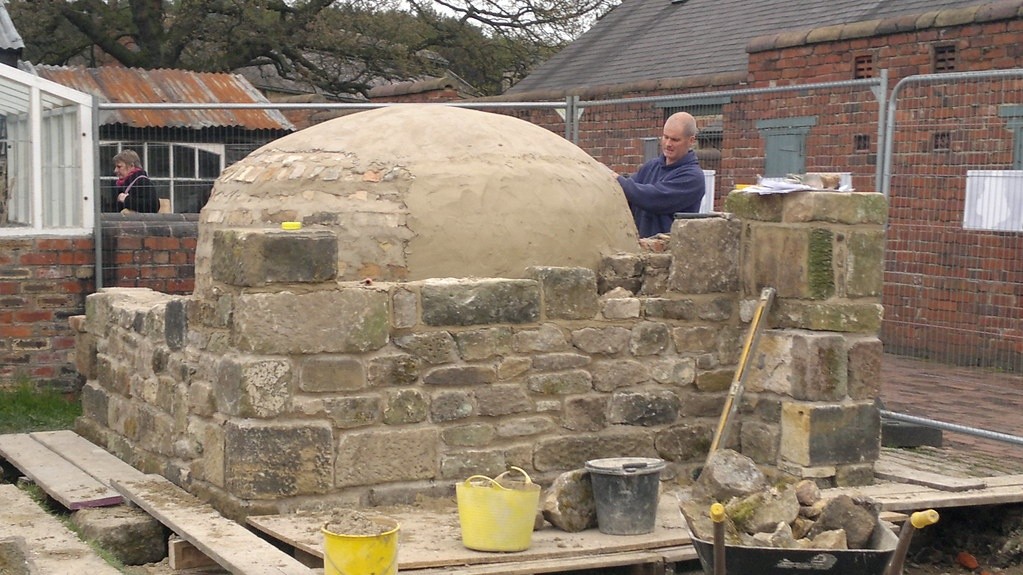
[686,517,940,575]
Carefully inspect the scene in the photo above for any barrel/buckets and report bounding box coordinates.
[321,516,401,575]
[455,466,541,551]
[585,456,666,535]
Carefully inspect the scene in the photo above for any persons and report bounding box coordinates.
[113,149,161,213]
[608,111,706,239]
